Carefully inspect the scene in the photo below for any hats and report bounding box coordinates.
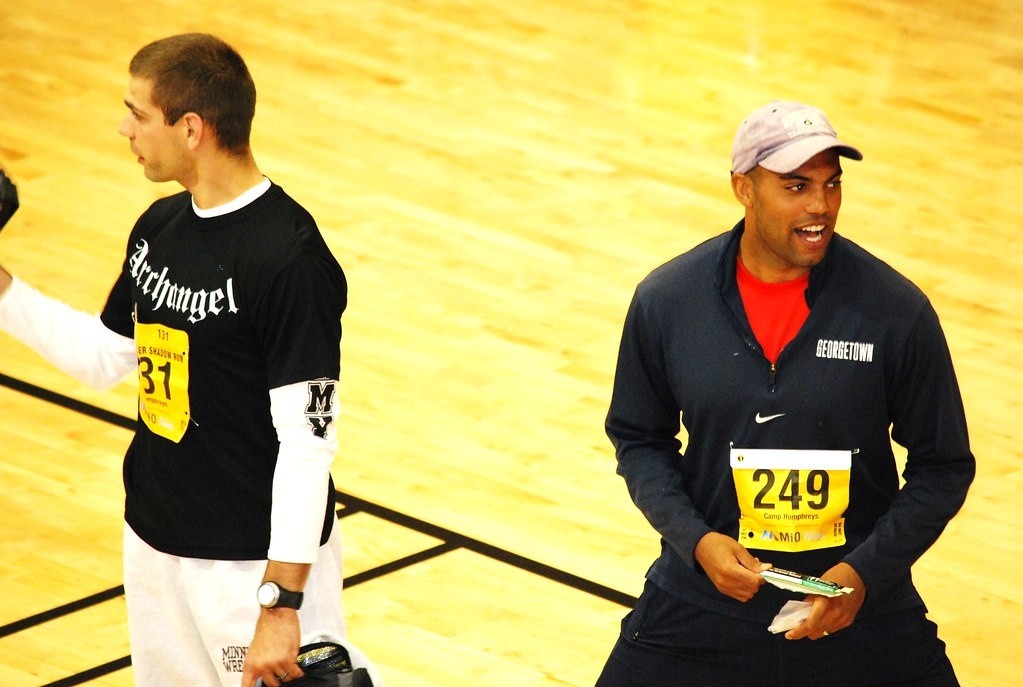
[729,99,863,175]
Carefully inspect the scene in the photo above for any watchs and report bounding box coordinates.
[257,581,304,610]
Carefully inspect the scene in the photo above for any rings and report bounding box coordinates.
[823,631,829,636]
[279,672,288,679]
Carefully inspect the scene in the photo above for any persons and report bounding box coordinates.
[0,34,347,687]
[594,104,975,687]
